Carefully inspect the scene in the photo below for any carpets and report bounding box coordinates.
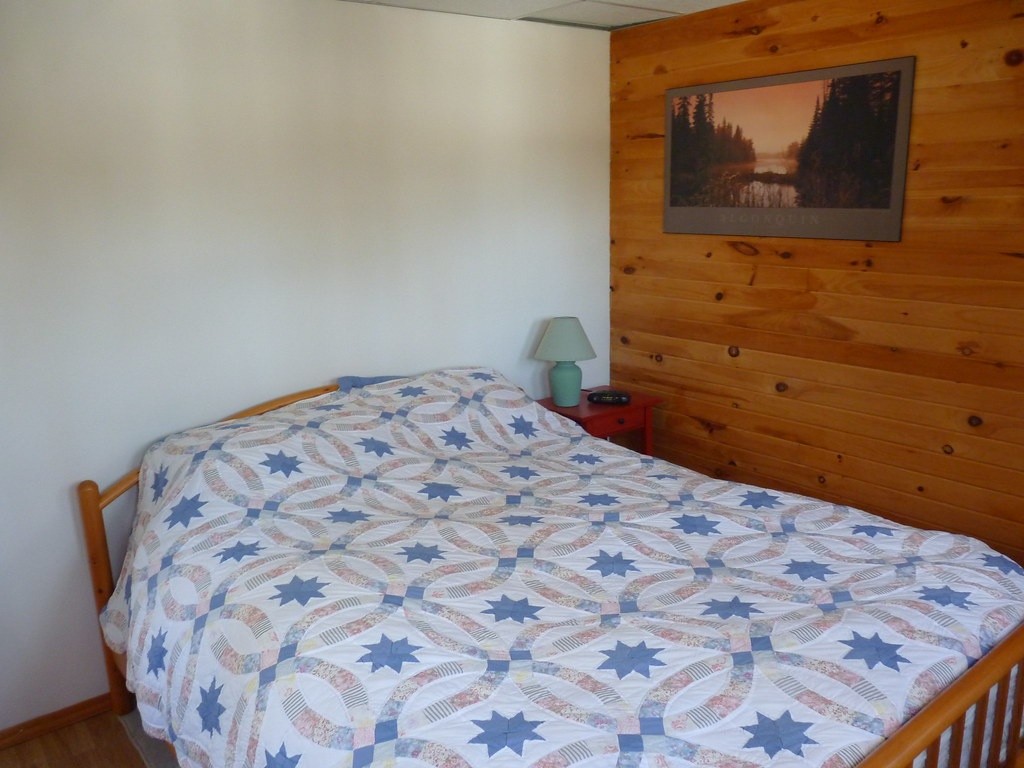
[118,702,181,768]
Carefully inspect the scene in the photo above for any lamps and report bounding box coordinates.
[533,317,597,408]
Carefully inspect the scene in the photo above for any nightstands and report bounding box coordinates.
[537,383,663,456]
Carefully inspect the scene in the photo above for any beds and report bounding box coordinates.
[74,365,1024,768]
